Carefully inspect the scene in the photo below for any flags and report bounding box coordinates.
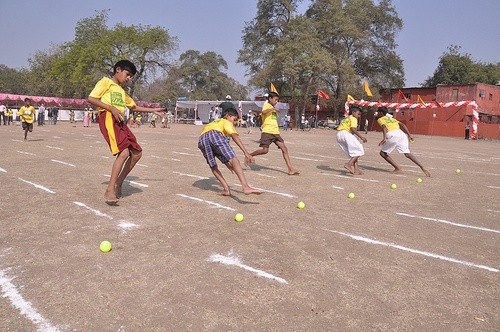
[348,95,354,101]
[318,90,329,99]
[417,96,426,105]
[364,80,373,97]
[271,83,279,95]
[400,90,408,103]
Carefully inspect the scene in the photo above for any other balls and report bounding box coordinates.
[416,177,422,183]
[235,213,243,222]
[455,169,461,172]
[100,240,111,252]
[390,183,398,189]
[410,139,413,144]
[298,201,305,209]
[348,192,355,199]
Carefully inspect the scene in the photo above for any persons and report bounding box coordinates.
[38,104,59,126]
[208,104,221,123]
[0,102,21,126]
[240,109,262,134]
[69,108,99,127]
[376,106,432,178]
[86,60,167,203]
[198,108,263,196]
[218,94,239,118]
[337,107,368,175]
[283,113,319,132]
[464,120,471,140]
[364,118,368,134]
[129,111,170,128]
[243,91,302,176]
[18,98,36,140]
